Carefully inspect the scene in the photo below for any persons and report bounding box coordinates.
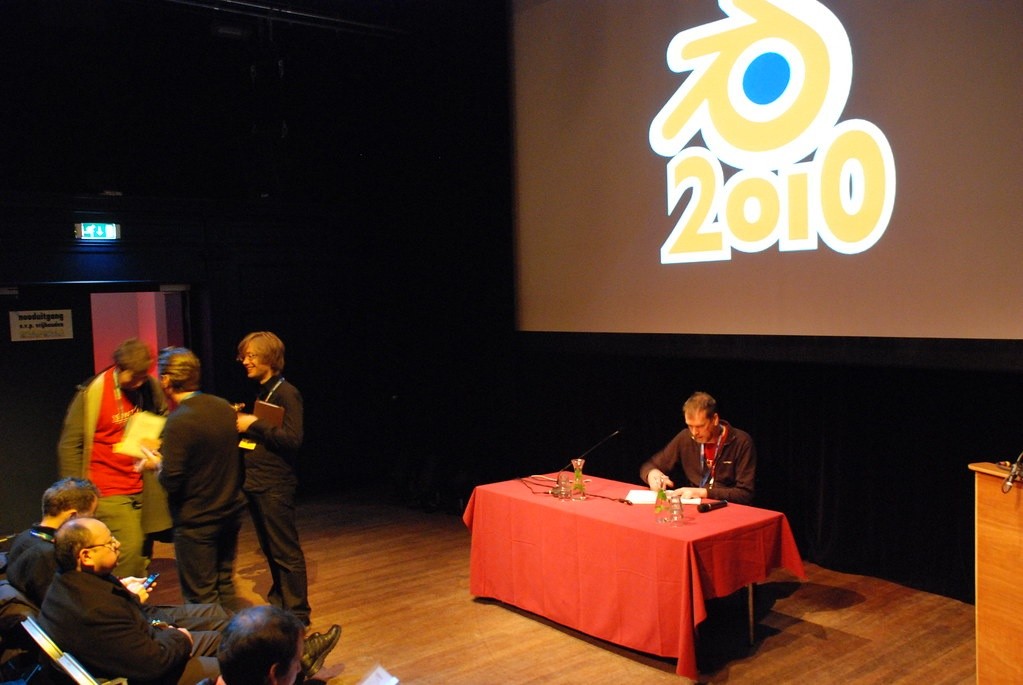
[8,477,231,633]
[38,517,339,685]
[142,347,238,618]
[58,338,170,580]
[197,606,343,685]
[639,392,755,505]
[234,332,312,621]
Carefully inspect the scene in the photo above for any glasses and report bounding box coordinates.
[83,536,117,552]
[236,353,258,361]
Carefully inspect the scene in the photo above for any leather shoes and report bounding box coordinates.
[300,624,342,677]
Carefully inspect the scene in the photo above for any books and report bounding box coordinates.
[254,400,285,429]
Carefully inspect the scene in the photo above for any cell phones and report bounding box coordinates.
[142,572,160,589]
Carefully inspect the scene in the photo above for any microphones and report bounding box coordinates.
[696,499,728,513]
[552,430,619,493]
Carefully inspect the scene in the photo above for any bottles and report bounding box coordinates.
[654,475,670,524]
[557,473,572,499]
[669,495,683,528]
[571,459,587,501]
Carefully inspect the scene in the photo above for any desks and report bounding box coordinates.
[469,471,784,681]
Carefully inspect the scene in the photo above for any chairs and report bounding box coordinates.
[0,532,128,685]
